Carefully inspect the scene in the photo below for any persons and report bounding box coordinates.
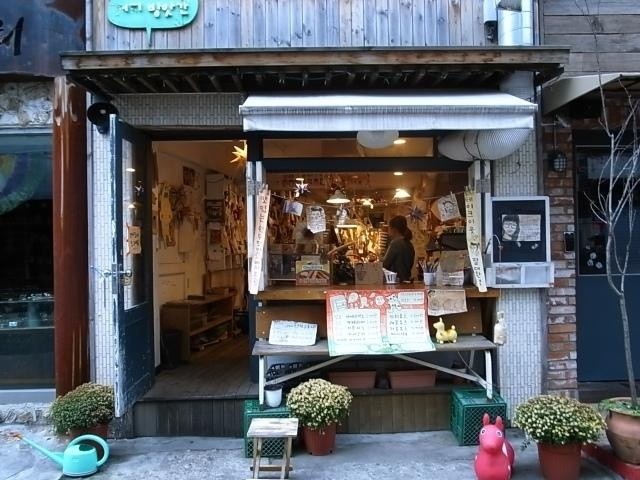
[502,213,520,241]
[380,215,415,286]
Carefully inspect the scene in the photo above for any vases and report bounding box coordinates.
[264,389,282,408]
[327,371,376,389]
[605,397,640,465]
[387,369,438,389]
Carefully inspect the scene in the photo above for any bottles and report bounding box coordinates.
[494,311,507,345]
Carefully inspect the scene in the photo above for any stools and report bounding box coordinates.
[246,418,299,480]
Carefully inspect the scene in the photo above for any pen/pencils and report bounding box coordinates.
[419,256,439,273]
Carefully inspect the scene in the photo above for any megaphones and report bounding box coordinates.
[87,101,120,127]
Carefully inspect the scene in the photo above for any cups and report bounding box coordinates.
[265,385,282,407]
[384,273,397,282]
[424,272,434,285]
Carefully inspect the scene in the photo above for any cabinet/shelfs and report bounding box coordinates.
[162,295,236,356]
[207,174,244,272]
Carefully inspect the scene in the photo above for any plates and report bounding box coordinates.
[384,282,398,284]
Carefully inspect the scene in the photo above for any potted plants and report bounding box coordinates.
[511,394,607,480]
[43,382,115,455]
[287,377,354,456]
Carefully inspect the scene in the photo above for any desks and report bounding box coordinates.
[251,335,497,405]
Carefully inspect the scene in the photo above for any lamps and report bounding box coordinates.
[325,185,350,204]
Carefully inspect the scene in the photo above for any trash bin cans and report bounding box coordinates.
[160,328,182,366]
